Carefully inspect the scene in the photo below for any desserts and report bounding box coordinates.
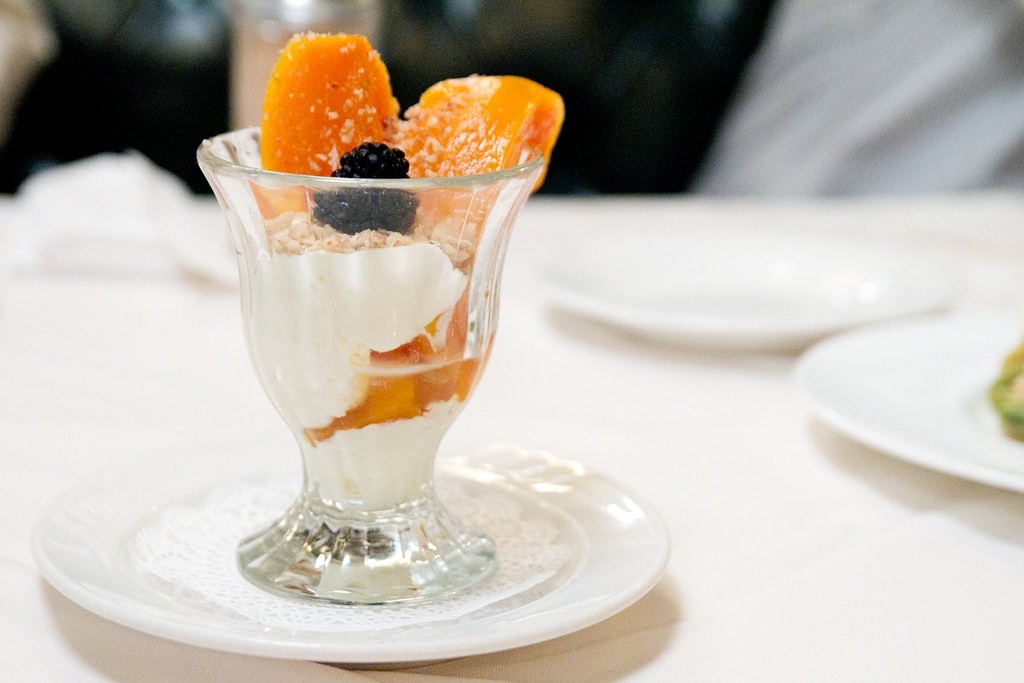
[243,33,564,512]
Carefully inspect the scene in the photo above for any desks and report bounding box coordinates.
[0,188,1024,683]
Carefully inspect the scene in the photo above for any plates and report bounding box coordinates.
[790,313,1024,493]
[29,438,672,665]
[534,241,945,354]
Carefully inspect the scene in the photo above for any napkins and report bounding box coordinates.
[19,151,236,297]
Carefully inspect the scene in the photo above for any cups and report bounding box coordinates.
[196,125,546,605]
